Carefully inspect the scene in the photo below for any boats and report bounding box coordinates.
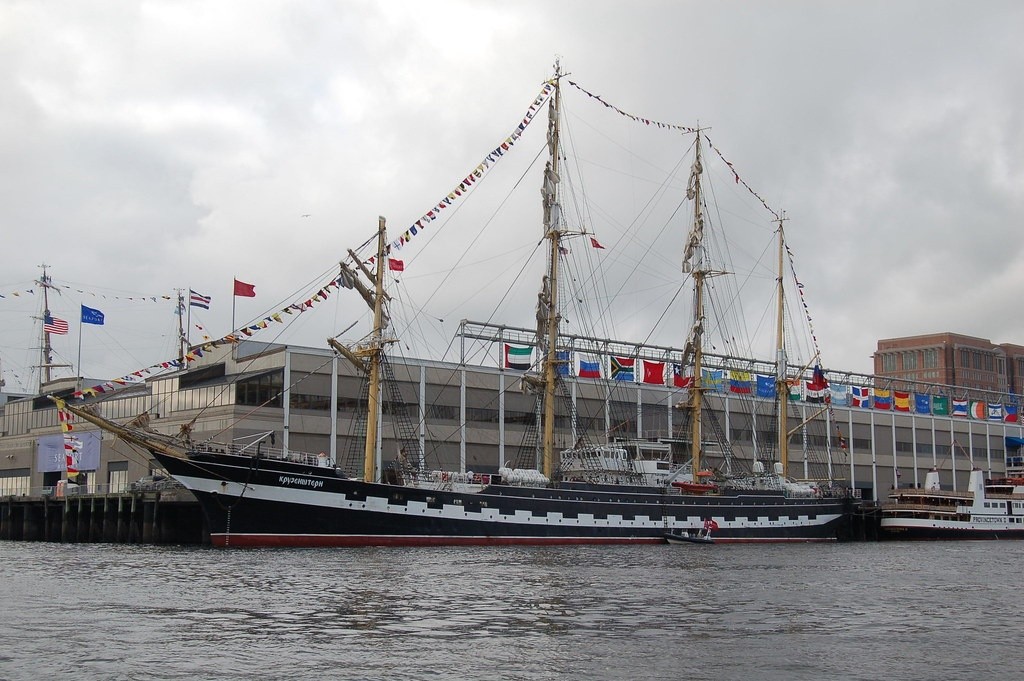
[662,531,711,542]
[672,481,719,495]
[879,439,1024,540]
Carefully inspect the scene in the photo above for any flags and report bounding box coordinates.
[388,258,404,271]
[805,381,826,403]
[80,304,105,326]
[893,390,911,412]
[1004,405,1018,422]
[987,402,1003,421]
[642,359,666,385]
[672,363,696,389]
[610,356,635,382]
[951,396,969,417]
[577,352,602,378]
[701,368,723,392]
[44,315,69,336]
[873,387,893,410]
[234,280,256,297]
[786,379,804,402]
[969,400,986,420]
[851,386,870,408]
[830,383,847,405]
[932,395,949,416]
[914,392,931,414]
[729,370,752,395]
[756,374,777,398]
[556,350,571,375]
[190,289,212,310]
[504,343,533,370]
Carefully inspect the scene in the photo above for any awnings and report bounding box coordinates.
[1005,436,1024,448]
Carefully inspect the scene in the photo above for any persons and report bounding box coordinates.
[395,449,419,485]
[773,459,783,477]
[752,458,765,477]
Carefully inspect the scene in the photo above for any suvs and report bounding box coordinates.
[134,477,166,490]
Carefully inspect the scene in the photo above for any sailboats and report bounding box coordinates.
[41,57,880,542]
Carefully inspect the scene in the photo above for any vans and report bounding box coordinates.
[41,487,56,495]
